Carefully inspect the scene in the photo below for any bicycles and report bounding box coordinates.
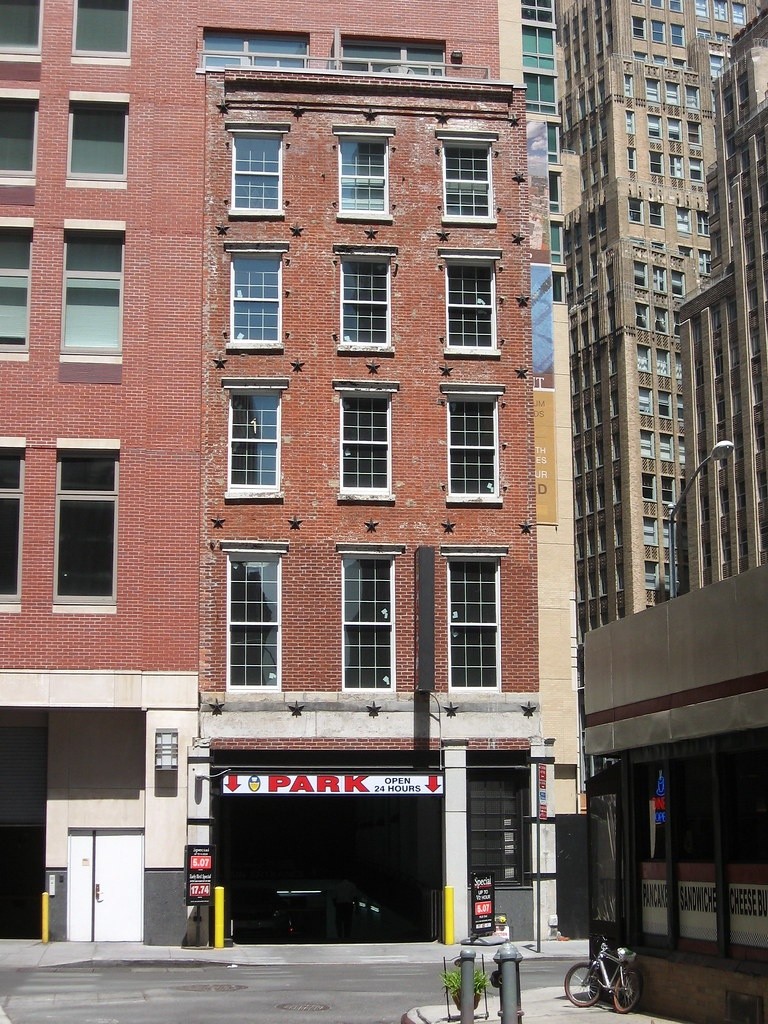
[563,932,646,1013]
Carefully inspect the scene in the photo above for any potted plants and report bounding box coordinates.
[438,968,490,1011]
